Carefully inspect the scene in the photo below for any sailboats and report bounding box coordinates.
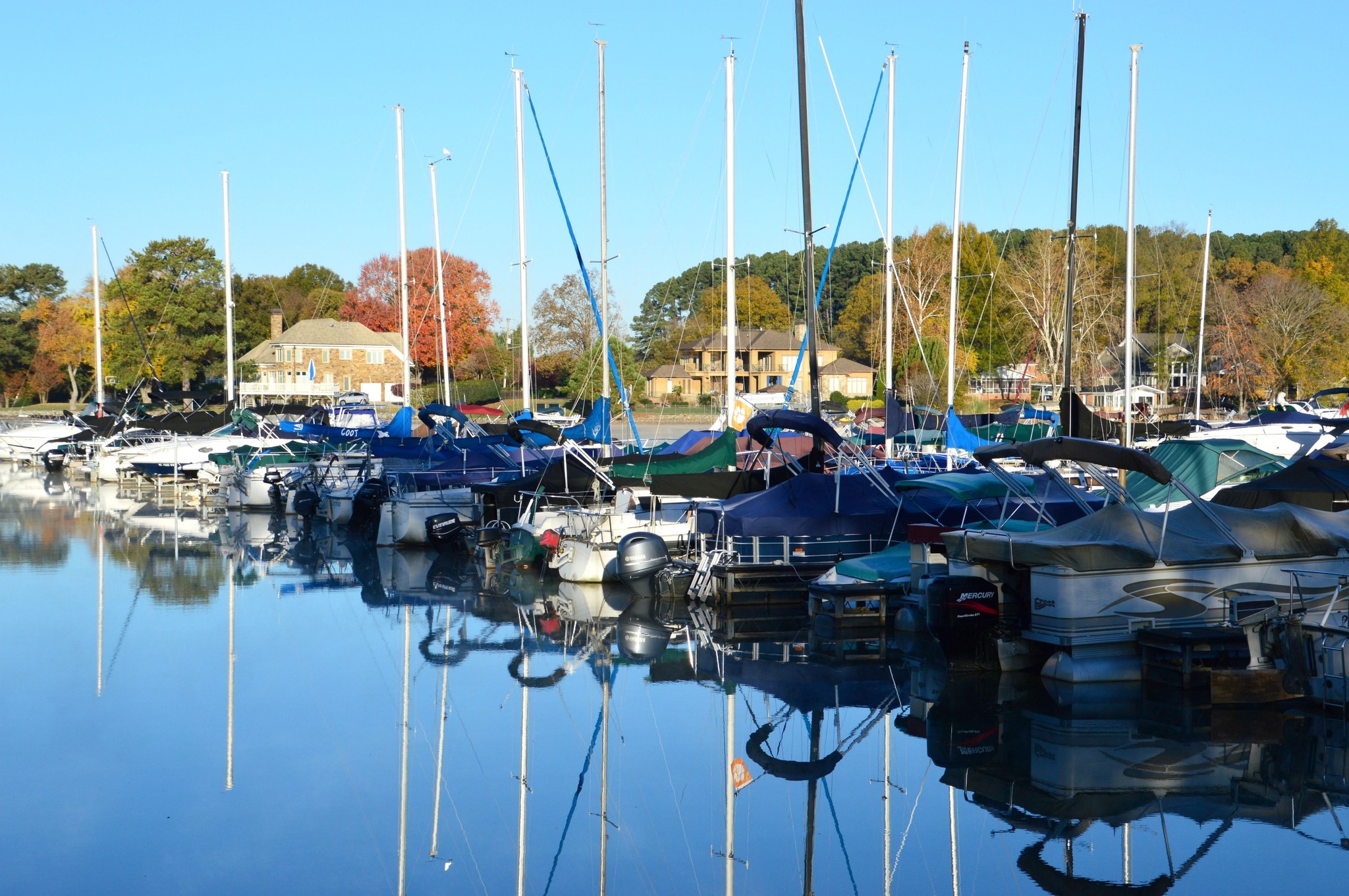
[1,1,1349,702]
